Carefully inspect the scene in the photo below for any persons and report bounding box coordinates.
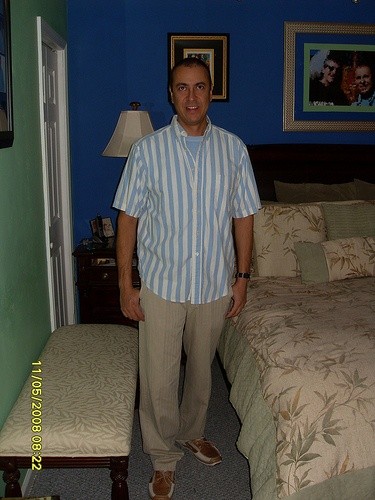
[112,58,262,500]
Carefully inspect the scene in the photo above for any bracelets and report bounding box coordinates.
[233,273,250,279]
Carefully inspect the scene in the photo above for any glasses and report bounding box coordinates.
[327,65,336,70]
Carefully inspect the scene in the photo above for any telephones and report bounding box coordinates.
[92,212,108,244]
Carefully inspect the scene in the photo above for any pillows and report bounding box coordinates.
[321,196,375,242]
[352,178,375,201]
[251,203,330,282]
[292,235,375,285]
[273,180,361,204]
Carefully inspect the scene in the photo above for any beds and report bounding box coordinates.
[216,132,375,500]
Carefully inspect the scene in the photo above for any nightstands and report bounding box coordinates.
[73,233,141,327]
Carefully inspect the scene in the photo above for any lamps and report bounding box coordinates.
[99,98,154,162]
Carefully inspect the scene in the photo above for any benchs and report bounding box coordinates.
[0,322,140,500]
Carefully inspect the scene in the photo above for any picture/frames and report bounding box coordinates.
[283,19,375,134]
[89,217,115,239]
[166,31,231,104]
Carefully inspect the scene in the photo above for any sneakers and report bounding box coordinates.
[149,470,176,500]
[176,435,221,467]
